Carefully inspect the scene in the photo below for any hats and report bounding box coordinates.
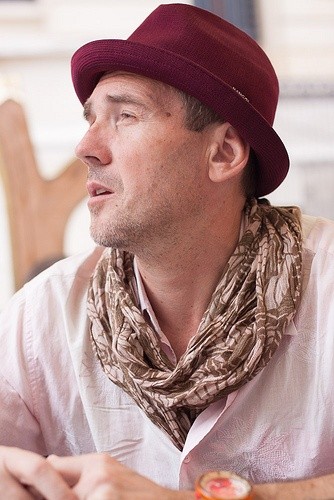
[70,2,291,197]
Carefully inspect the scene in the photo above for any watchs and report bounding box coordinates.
[193,469,255,500]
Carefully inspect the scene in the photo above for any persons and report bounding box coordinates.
[0,4,334,500]
